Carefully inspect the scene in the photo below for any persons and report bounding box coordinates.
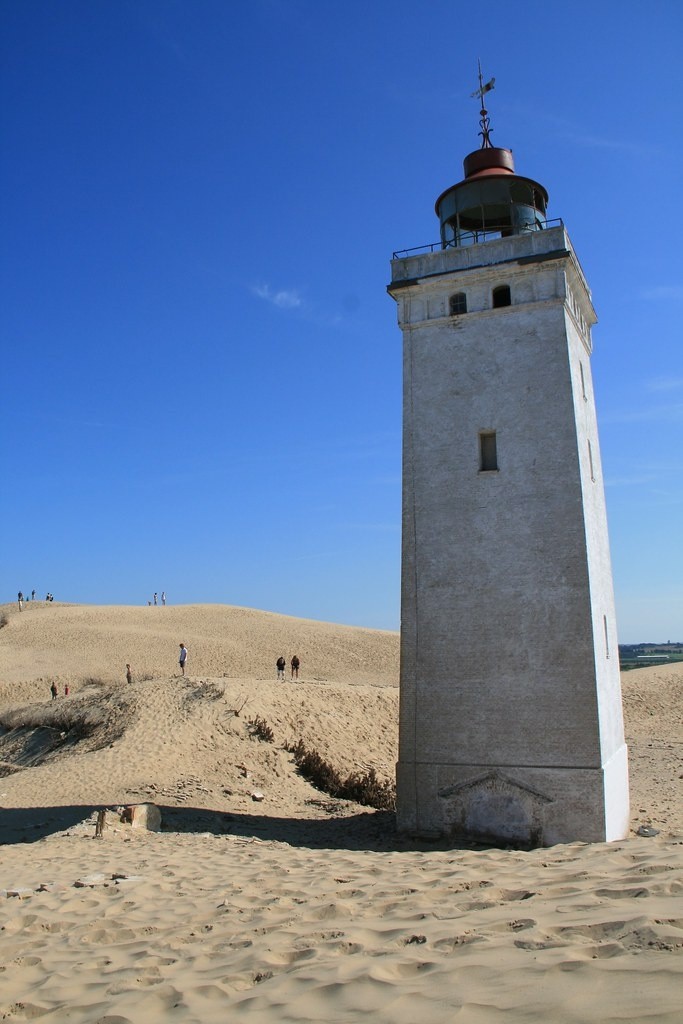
[51,681,58,699]
[161,592,166,605]
[291,655,300,681]
[179,643,188,675]
[154,593,158,606]
[126,664,132,684]
[276,657,286,680]
[18,589,53,602]
[65,684,70,696]
